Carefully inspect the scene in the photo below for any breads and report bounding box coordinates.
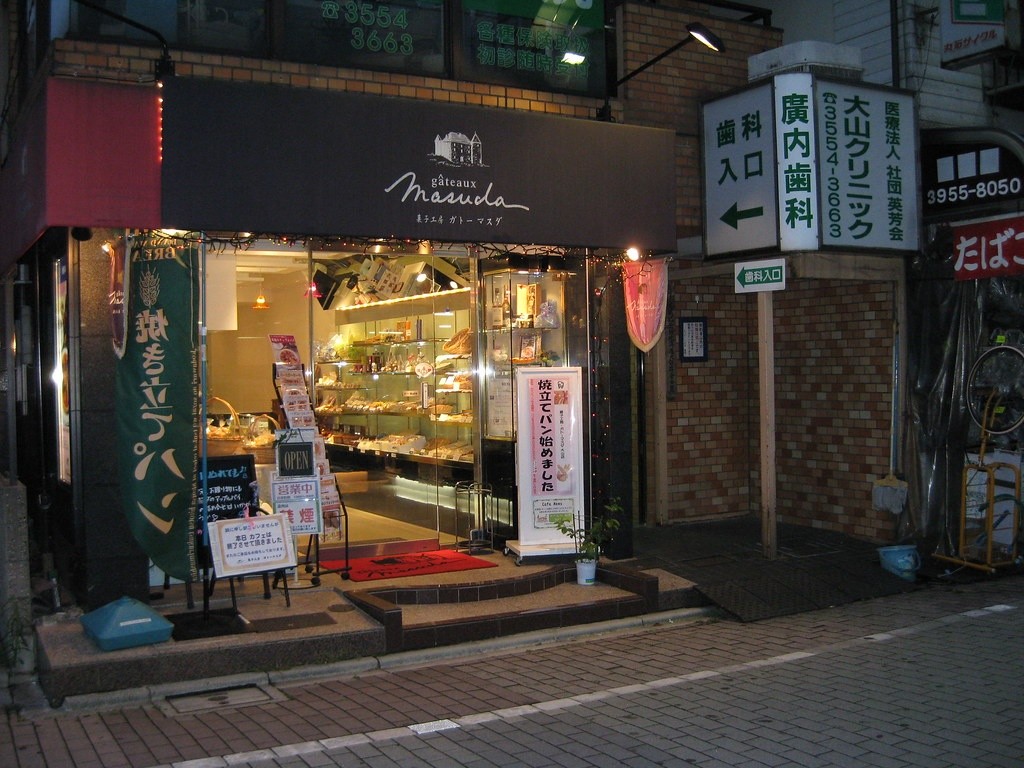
[206,425,228,438]
[253,432,276,447]
[423,437,474,461]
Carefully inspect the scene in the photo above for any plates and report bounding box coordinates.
[278,349,299,369]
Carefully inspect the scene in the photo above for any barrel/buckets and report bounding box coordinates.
[878,546,920,582]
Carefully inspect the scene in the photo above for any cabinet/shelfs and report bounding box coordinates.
[311,252,594,537]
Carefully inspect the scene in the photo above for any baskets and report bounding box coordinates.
[443,328,472,353]
[199,397,242,457]
[242,414,281,464]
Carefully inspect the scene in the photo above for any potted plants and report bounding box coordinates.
[556,497,623,585]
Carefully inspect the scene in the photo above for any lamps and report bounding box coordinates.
[600,21,725,122]
[252,268,270,309]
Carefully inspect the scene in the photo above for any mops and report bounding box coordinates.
[870,318,910,514]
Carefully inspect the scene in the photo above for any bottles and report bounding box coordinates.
[376,353,380,372]
[502,285,513,328]
[492,288,503,329]
[380,352,384,371]
[371,353,376,363]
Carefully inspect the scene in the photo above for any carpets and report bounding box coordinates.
[318,548,498,581]
